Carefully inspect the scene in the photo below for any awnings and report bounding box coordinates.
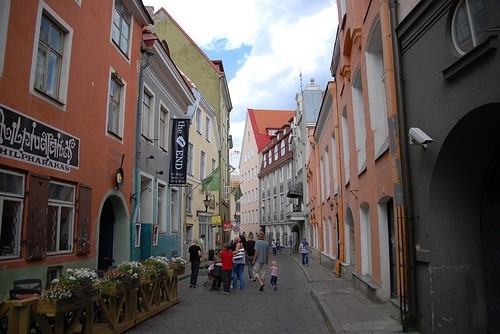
[287,182,303,198]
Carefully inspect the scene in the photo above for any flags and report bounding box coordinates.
[233,186,243,202]
[201,166,221,191]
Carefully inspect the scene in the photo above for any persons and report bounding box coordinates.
[188,231,281,294]
[288,238,292,255]
[299,238,310,266]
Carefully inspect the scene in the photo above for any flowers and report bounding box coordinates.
[34,268,103,302]
[101,254,188,284]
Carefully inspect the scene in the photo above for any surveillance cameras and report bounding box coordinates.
[409,127,435,149]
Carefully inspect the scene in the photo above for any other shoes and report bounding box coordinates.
[230,285,234,289]
[249,277,252,278]
[189,285,192,288]
[231,277,239,280]
[259,284,265,291]
[273,285,277,291]
[193,285,196,288]
[222,290,230,294]
[251,278,256,282]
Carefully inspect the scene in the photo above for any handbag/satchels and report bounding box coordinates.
[233,243,244,260]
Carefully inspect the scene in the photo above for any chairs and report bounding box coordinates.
[9,278,42,299]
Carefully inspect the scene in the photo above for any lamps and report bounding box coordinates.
[196,194,211,216]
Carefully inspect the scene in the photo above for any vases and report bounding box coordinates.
[102,268,185,296]
[36,288,101,313]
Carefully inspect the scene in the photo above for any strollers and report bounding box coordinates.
[203,262,222,291]
